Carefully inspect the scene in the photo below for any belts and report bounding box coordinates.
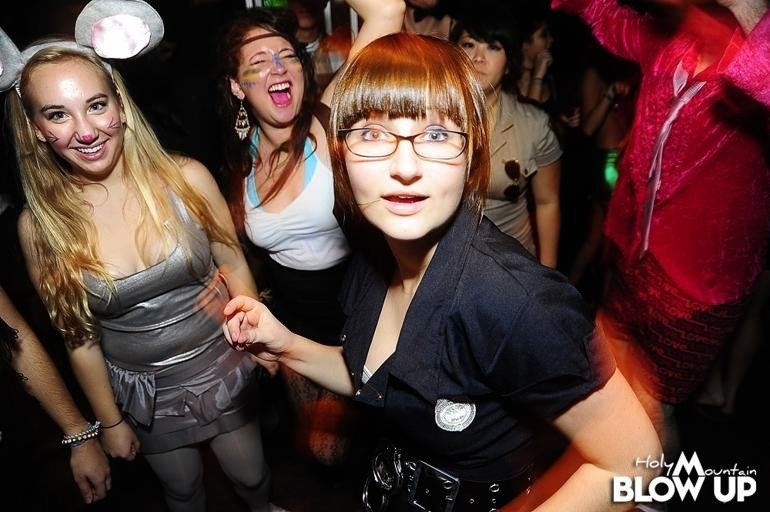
[359,446,540,512]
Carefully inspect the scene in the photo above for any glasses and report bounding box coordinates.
[341,128,470,159]
